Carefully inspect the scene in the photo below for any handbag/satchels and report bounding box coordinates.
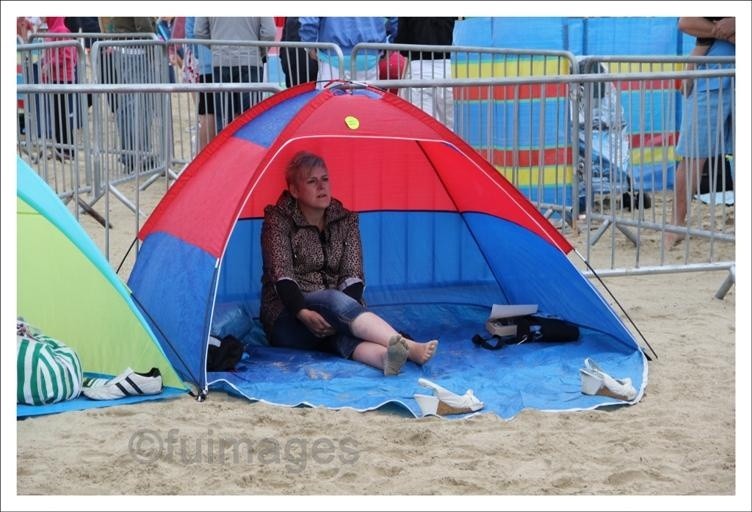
[472,314,580,351]
[17,316,83,405]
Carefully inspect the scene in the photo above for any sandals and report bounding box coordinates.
[413,377,485,416]
[579,357,637,401]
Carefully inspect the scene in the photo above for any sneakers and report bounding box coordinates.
[83,367,163,400]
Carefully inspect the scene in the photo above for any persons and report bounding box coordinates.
[16,15,462,169]
[678,15,736,99]
[663,16,736,248]
[259,151,441,380]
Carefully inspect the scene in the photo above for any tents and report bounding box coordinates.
[115,77,659,420]
[448,17,731,213]
[15,141,205,422]
